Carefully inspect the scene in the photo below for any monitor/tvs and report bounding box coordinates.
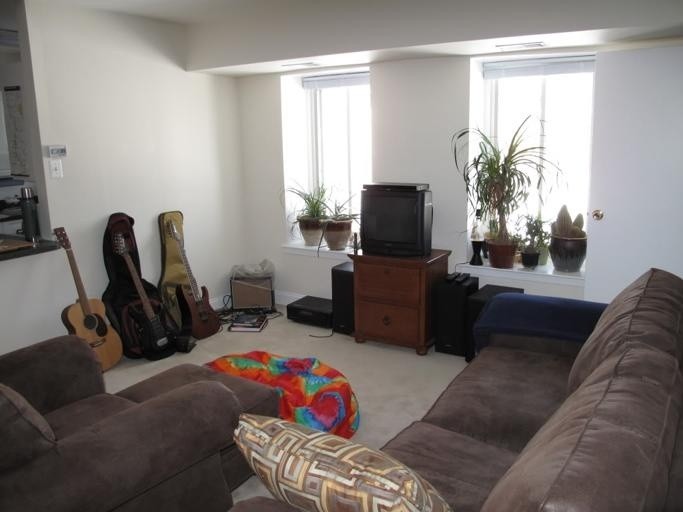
[360,189,434,258]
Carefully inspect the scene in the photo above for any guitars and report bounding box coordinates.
[51,219,221,373]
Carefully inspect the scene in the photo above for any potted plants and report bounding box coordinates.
[516,211,542,268]
[316,192,361,258]
[546,200,587,274]
[445,111,568,269]
[280,182,333,247]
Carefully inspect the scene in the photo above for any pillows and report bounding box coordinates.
[228,409,457,511]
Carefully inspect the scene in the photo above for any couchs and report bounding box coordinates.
[217,252,683,512]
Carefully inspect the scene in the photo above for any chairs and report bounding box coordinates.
[0,321,250,512]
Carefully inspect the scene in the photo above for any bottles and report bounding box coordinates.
[468,210,484,265]
[20,186,39,242]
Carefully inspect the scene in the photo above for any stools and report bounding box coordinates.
[114,363,279,489]
[197,341,365,439]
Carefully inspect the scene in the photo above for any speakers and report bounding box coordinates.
[465,285,524,362]
[432,277,479,355]
[331,262,356,334]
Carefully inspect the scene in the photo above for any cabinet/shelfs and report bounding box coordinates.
[345,248,449,359]
